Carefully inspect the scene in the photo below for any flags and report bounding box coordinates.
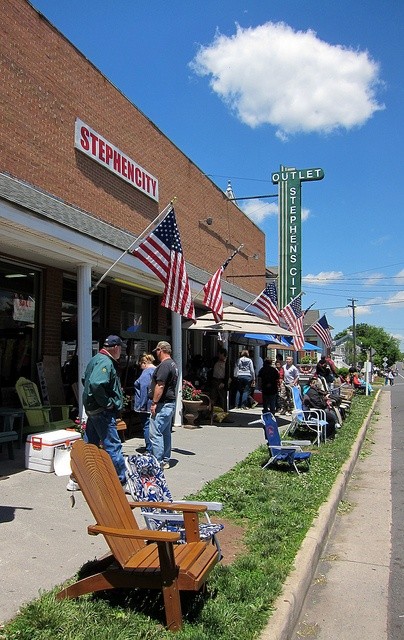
[278,294,305,351]
[250,280,280,326]
[310,314,334,349]
[129,208,197,325]
[202,248,239,323]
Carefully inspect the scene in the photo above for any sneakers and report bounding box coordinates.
[335,423,341,429]
[67,473,81,491]
[163,461,170,469]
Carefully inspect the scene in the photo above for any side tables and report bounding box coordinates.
[0,409,27,451]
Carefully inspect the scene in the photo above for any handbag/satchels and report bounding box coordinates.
[54,444,72,477]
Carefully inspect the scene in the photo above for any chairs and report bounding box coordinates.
[56,438,219,630]
[15,376,76,432]
[123,451,223,560]
[72,382,127,443]
[282,385,328,447]
[248,411,312,475]
[183,380,213,425]
[303,375,364,420]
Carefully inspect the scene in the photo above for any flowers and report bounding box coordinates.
[182,379,201,401]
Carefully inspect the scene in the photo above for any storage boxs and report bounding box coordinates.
[23,429,81,473]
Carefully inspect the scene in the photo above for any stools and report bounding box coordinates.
[0,431,18,460]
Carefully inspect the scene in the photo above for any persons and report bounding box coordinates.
[342,361,397,392]
[315,359,334,389]
[213,348,229,411]
[305,378,336,438]
[274,360,287,415]
[147,340,178,470]
[66,334,130,491]
[232,350,256,408]
[282,356,300,416]
[256,358,280,415]
[131,352,158,453]
[247,365,258,408]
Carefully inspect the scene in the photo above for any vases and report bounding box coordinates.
[182,400,203,429]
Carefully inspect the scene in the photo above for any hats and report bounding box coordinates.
[152,341,170,352]
[103,335,127,348]
